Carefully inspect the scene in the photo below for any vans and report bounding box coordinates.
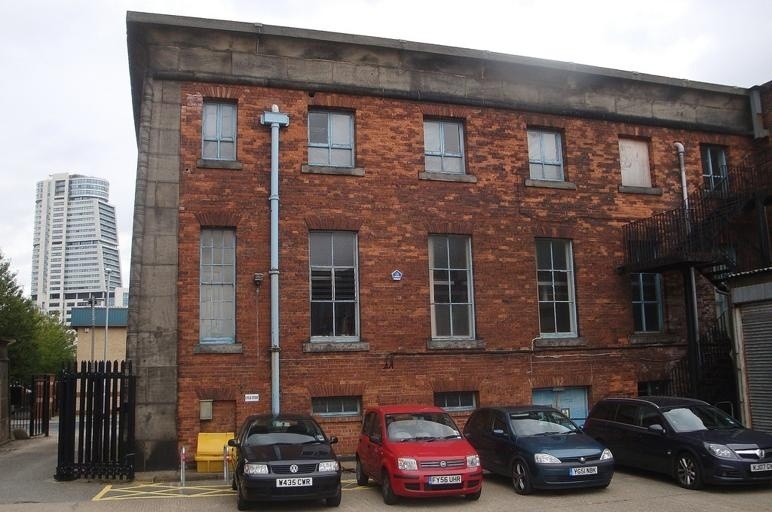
[354,402,482,505]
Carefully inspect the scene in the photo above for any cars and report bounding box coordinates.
[583,394,772,493]
[461,404,616,496]
[226,412,343,510]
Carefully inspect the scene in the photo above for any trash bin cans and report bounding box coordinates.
[194,431,238,474]
